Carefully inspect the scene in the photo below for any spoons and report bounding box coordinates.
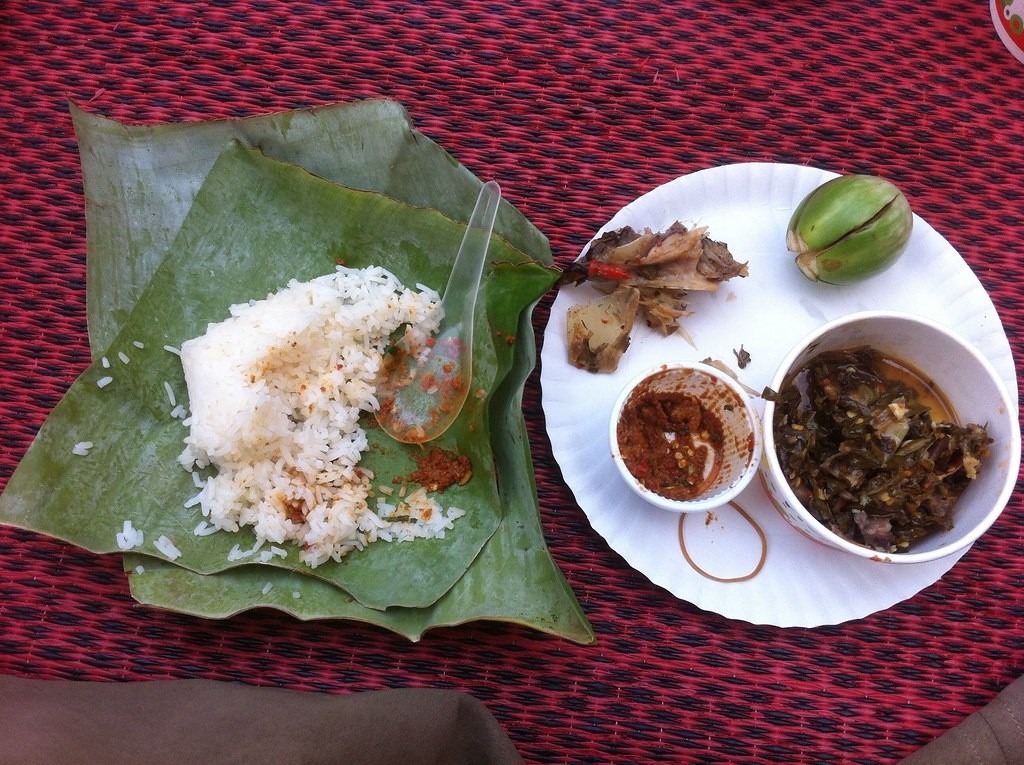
[373,180,502,446]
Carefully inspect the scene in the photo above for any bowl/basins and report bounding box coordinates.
[759,310,1022,565]
[610,363,761,513]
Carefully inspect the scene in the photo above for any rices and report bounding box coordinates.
[70,264,466,566]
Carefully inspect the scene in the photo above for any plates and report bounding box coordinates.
[539,162,1019,631]
